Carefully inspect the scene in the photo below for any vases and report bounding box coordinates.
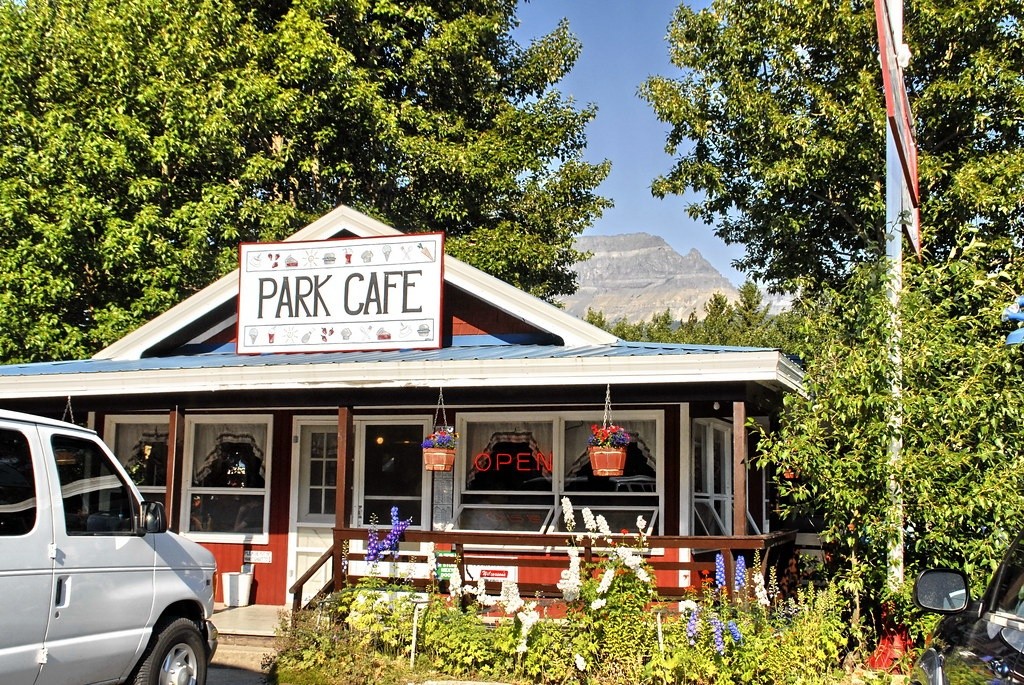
[782,468,801,478]
[589,446,628,477]
[422,448,457,473]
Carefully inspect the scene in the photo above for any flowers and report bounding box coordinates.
[588,423,631,449]
[422,429,459,449]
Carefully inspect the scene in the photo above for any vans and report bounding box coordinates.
[0,408,220,685]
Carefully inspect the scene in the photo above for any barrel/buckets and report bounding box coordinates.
[221,571,253,607]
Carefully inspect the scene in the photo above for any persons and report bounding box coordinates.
[380,442,553,531]
[190,494,265,532]
[834,523,868,569]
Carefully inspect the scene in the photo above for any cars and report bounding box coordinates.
[911,519,1024,685]
[606,473,656,491]
